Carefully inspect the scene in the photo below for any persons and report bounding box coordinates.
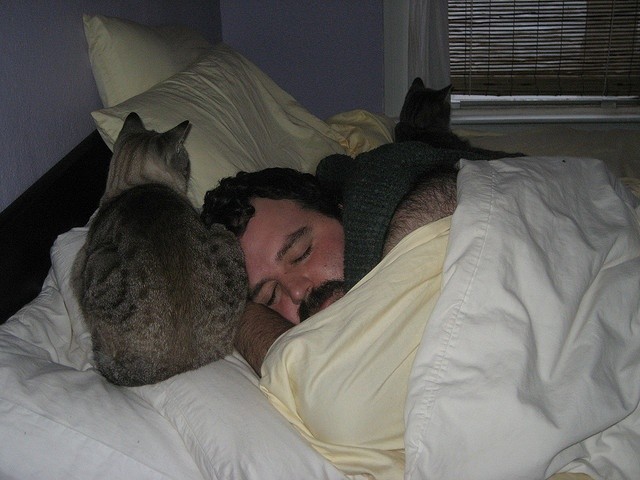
[199,167,457,377]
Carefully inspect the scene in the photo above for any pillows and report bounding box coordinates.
[78,12,214,107]
[88,41,345,222]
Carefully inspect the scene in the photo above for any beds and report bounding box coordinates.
[1,123,640,480]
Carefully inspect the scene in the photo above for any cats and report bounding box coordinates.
[394,77,528,160]
[71,111,250,387]
